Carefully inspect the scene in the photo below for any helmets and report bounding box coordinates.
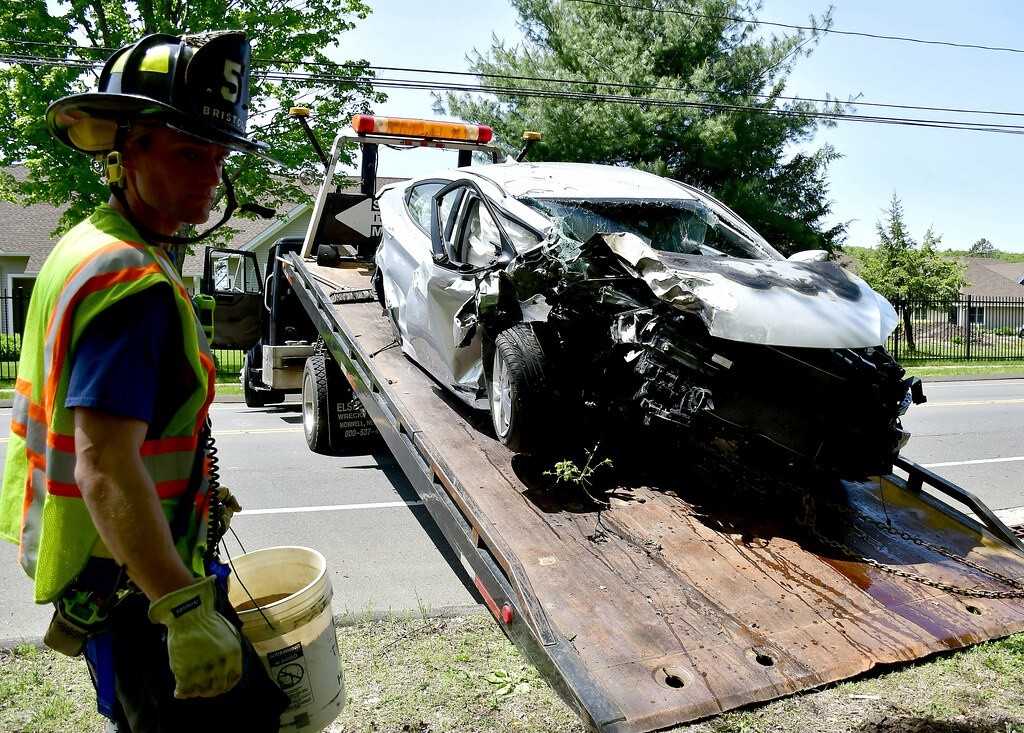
[47,28,274,163]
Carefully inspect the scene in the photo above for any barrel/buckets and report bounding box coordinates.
[219,524,346,733]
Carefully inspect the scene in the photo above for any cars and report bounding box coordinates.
[373,161,928,494]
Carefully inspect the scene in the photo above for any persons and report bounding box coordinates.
[0,29,291,733]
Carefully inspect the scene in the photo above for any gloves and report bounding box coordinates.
[147,574,239,700]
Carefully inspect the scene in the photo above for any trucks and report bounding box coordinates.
[195,110,1024,733]
